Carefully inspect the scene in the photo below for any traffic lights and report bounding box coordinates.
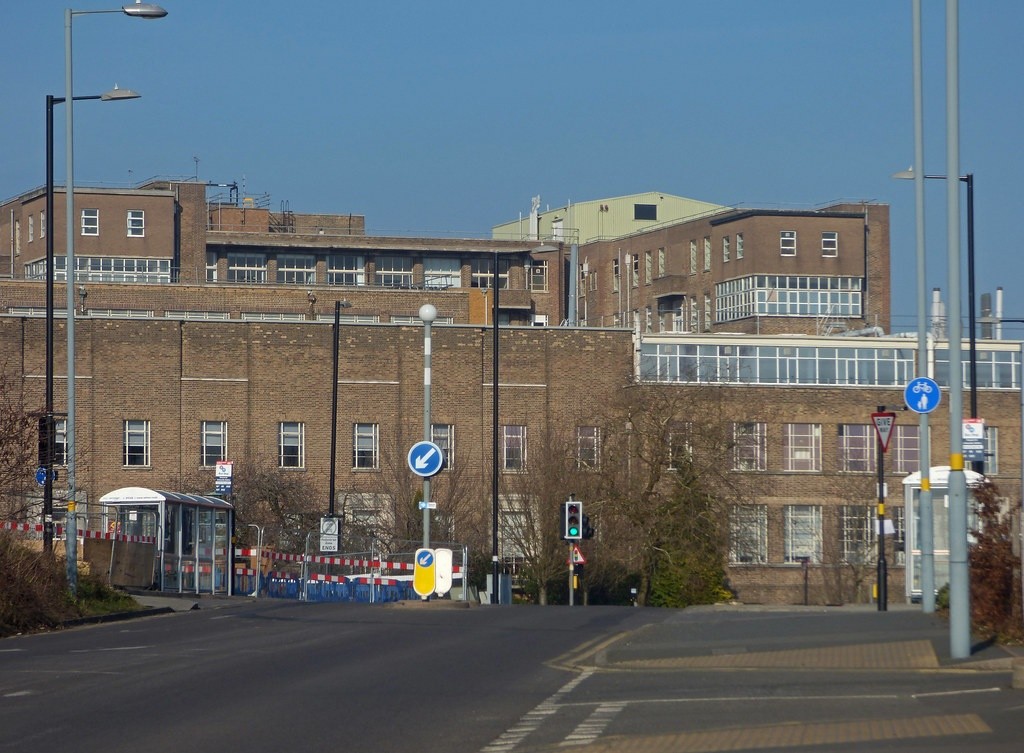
[564,500,583,541]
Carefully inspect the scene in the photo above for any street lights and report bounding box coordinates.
[892,166,981,476]
[62,0,171,603]
[41,86,143,566]
[419,304,438,601]
